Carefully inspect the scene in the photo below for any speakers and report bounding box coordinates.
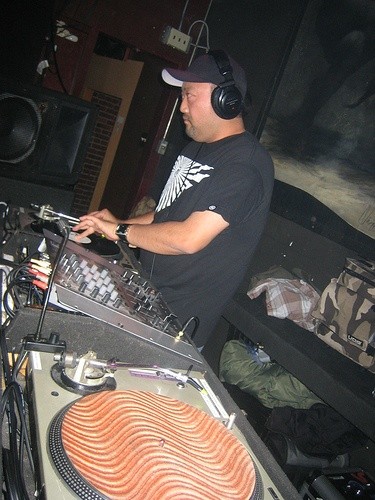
[0,78,99,186]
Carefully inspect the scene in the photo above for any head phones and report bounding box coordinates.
[208,48,243,120]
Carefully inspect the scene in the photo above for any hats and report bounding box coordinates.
[161,51,248,100]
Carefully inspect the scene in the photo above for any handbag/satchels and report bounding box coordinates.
[310,256,375,375]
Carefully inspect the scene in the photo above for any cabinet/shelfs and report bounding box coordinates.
[203,292,375,500]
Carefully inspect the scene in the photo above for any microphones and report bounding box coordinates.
[35,221,71,341]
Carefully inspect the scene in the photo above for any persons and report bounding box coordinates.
[72,48,276,355]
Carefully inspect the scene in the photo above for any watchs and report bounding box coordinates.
[114,222,134,243]
